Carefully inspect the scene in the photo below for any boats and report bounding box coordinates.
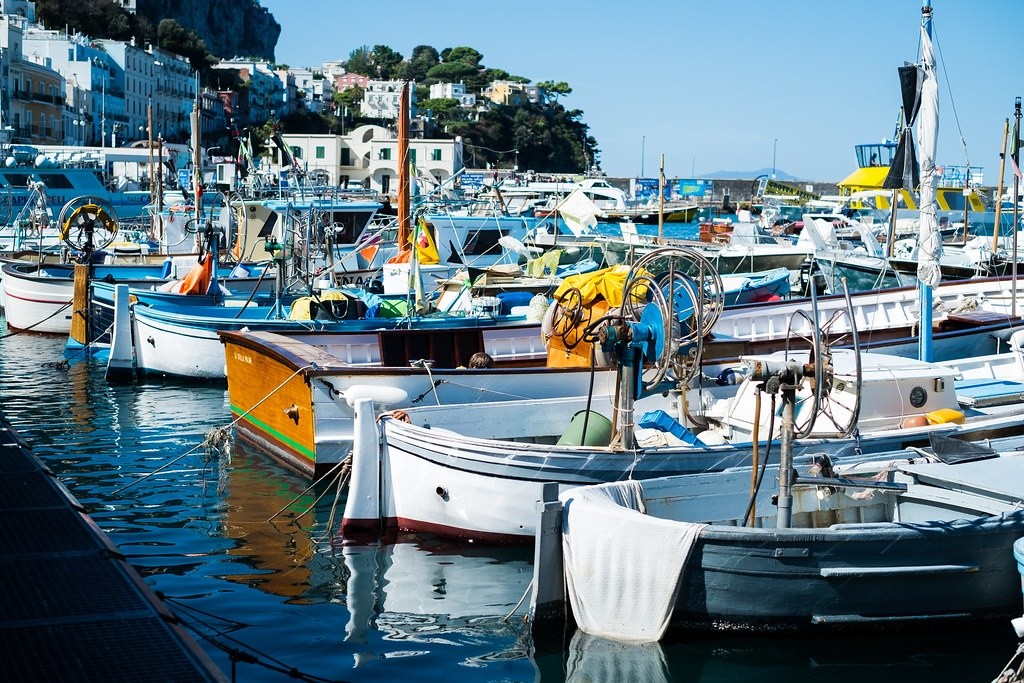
[523,623,1020,683]
[0,0,1024,543]
[334,532,541,636]
[220,435,352,609]
[528,264,1024,639]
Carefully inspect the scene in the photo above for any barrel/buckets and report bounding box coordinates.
[927,409,965,425]
[556,409,613,446]
[497,292,535,315]
[472,297,501,316]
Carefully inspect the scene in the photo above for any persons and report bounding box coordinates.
[869,153,879,167]
